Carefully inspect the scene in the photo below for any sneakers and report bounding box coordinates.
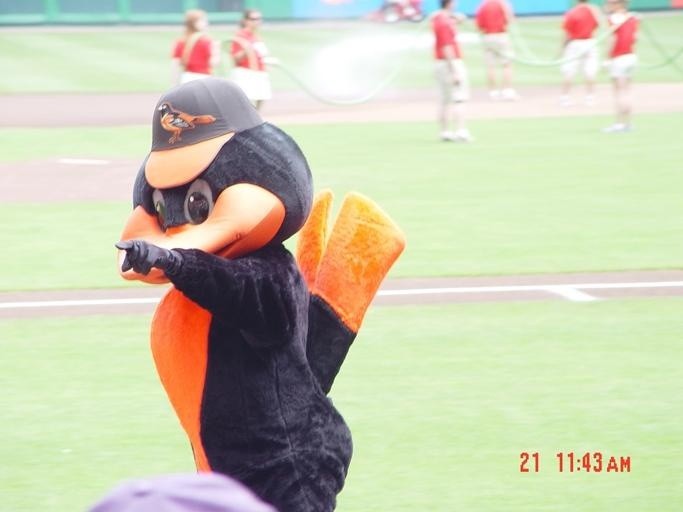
[606,124,630,131]
[561,96,592,106]
[492,89,515,100]
[438,132,470,142]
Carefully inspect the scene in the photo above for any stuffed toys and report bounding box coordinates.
[112,74,407,511]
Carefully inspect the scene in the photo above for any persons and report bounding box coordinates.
[427,1,472,144]
[475,2,519,102]
[559,1,605,107]
[228,9,282,112]
[171,8,218,84]
[600,1,644,133]
